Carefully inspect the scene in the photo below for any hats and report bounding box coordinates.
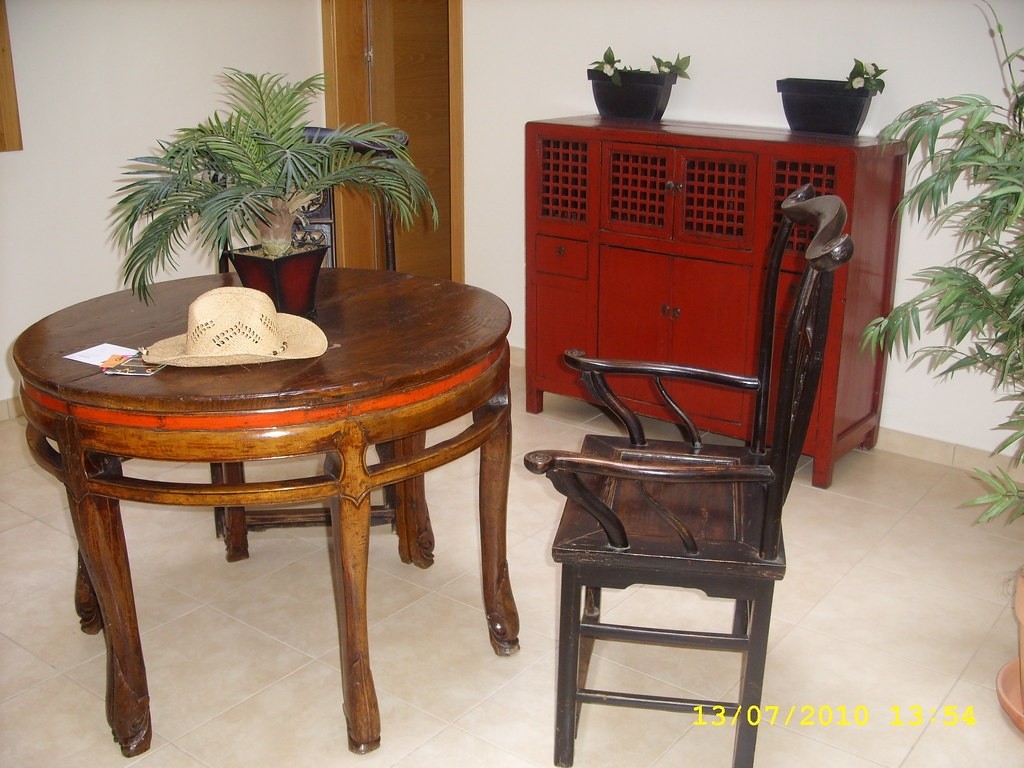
[142,286,329,368]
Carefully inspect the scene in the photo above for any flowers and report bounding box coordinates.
[588,48,690,87]
[845,58,889,96]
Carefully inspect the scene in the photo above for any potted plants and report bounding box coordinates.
[858,0,1024,733]
[105,65,440,323]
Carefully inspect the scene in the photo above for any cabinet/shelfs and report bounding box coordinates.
[523,113,908,489]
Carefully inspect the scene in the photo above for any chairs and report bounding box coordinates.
[196,125,413,565]
[524,178,858,768]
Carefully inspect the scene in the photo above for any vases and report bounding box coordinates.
[777,78,877,137]
[587,69,677,122]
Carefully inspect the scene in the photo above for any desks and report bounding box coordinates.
[12,267,521,758]
